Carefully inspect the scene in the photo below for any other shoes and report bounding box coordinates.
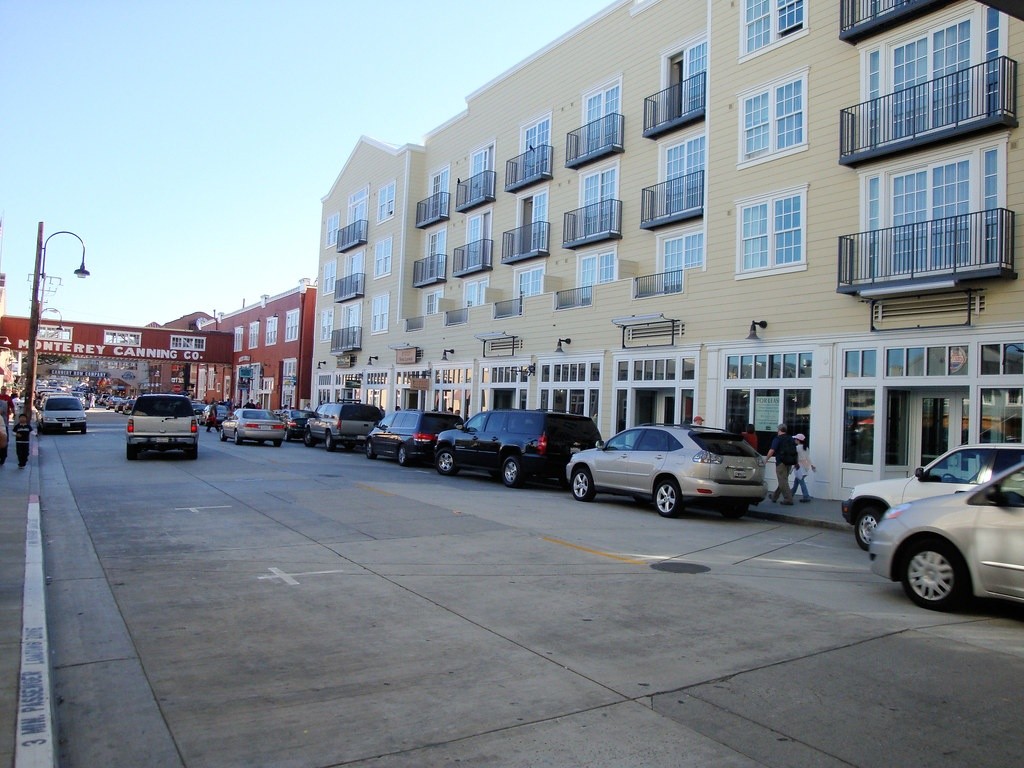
[800,498,810,502]
[768,494,776,503]
[780,501,792,506]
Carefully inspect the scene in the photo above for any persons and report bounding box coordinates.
[395,406,401,412]
[304,402,312,410]
[282,405,288,410]
[185,393,262,433]
[765,423,800,505]
[741,424,759,451]
[683,420,691,428]
[88,391,96,408]
[690,416,704,432]
[790,434,815,503]
[0,386,32,466]
[448,408,460,415]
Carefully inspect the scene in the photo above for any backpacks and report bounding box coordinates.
[777,435,798,465]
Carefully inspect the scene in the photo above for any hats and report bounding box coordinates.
[694,416,704,422]
[777,424,787,431]
[792,434,805,441]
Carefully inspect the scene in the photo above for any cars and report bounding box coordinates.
[190,402,206,424]
[867,457,1024,612]
[34,381,90,435]
[199,404,233,429]
[89,391,133,414]
[220,409,286,446]
[841,439,1024,552]
[278,410,316,441]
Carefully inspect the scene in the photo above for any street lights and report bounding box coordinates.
[24,230,91,423]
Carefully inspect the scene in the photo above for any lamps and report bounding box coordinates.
[366,356,378,366]
[555,338,571,352]
[316,361,327,370]
[441,349,456,362]
[748,320,768,340]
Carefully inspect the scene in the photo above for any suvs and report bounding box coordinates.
[304,399,383,453]
[566,425,767,517]
[434,409,604,488]
[127,392,202,460]
[364,408,464,465]
